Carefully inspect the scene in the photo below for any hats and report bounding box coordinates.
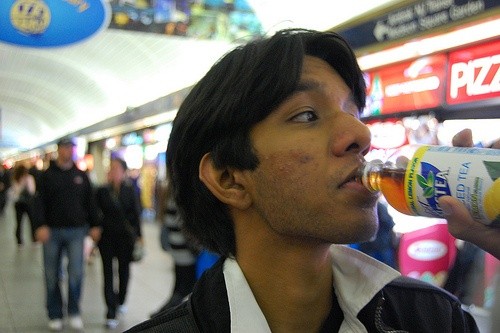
[57,137,76,147]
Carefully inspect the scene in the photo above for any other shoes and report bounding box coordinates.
[67,312,83,330]
[48,318,63,331]
[105,304,127,332]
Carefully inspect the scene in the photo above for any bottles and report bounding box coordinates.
[355,143,500,227]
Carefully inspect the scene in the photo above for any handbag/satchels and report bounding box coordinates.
[122,235,143,261]
[19,182,33,206]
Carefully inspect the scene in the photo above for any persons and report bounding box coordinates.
[33,135,102,328]
[93,149,144,331]
[0,145,500,306]
[121,27,500,333]
